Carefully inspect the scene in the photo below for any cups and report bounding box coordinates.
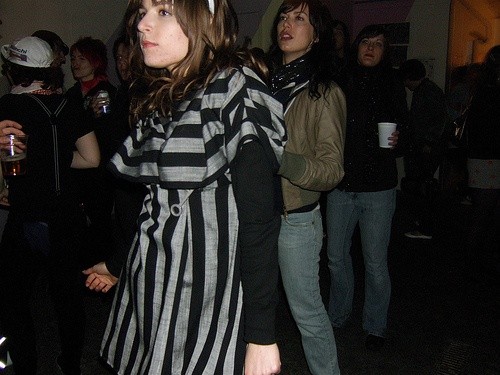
[0,135,27,176]
[378,122,397,147]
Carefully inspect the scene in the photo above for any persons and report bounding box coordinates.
[82,0,287,375]
[0,0,500,375]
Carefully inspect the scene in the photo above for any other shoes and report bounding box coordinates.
[367,333,385,352]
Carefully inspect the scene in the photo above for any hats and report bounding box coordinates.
[1,36,56,68]
